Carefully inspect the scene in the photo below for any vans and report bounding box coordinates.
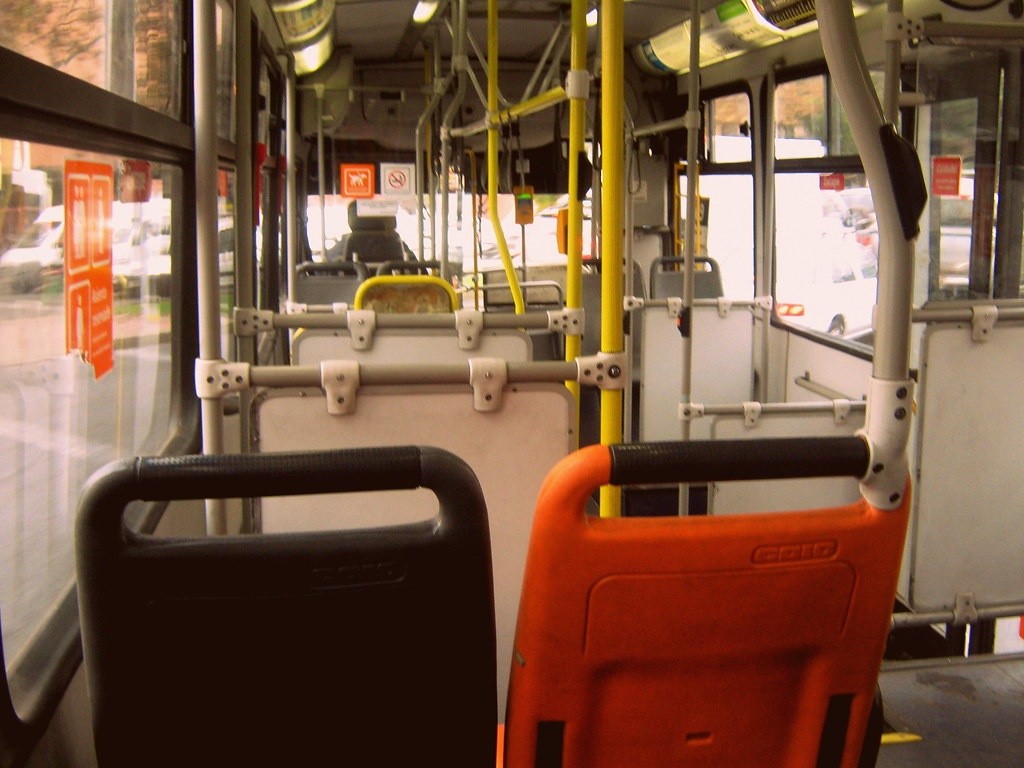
[0,192,255,295]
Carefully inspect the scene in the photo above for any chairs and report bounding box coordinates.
[344,200,407,262]
[68,449,503,768]
[578,254,726,375]
[298,261,459,316]
[498,434,914,763]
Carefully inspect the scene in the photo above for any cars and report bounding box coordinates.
[451,117,1011,366]
